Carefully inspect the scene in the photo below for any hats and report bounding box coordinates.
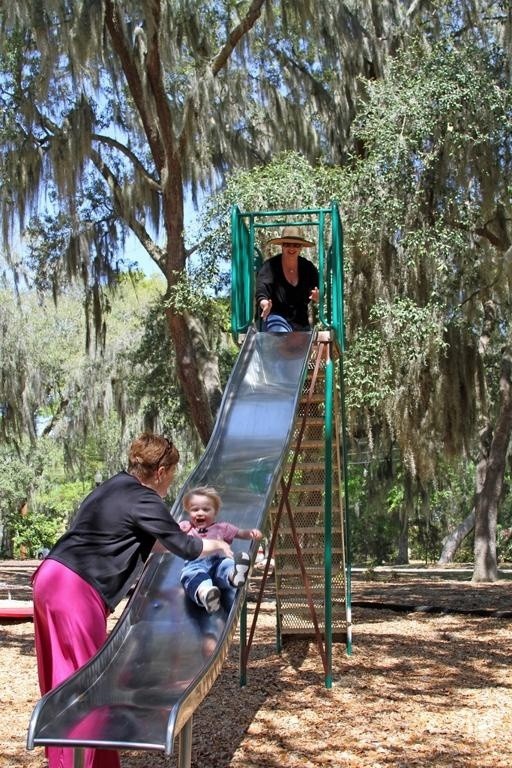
[266,226,315,247]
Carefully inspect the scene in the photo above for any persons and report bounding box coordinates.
[31,433,235,768]
[255,226,325,333]
[178,484,262,614]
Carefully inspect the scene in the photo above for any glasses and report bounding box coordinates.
[158,438,173,465]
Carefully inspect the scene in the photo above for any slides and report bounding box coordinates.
[27,323,318,758]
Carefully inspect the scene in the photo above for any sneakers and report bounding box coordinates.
[203,585,221,615]
[231,551,250,588]
